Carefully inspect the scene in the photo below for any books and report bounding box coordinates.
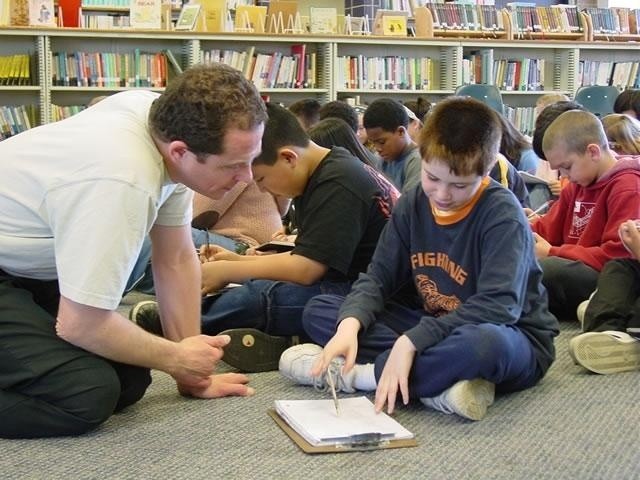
[462,49,545,91]
[0,102,89,143]
[504,104,538,138]
[339,95,360,107]
[338,55,435,91]
[1,52,184,87]
[371,8,409,37]
[578,60,639,87]
[201,44,315,89]
[1,1,228,34]
[233,2,338,34]
[424,4,639,35]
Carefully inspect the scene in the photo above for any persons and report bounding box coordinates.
[498,113,539,177]
[535,89,639,142]
[404,96,433,144]
[353,105,380,156]
[289,96,358,142]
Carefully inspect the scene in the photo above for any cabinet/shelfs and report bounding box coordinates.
[336,37,461,111]
[461,38,578,139]
[1,27,45,144]
[55,2,446,37]
[504,7,588,42]
[46,31,196,127]
[582,7,640,42]
[196,34,335,106]
[414,7,510,41]
[577,42,640,100]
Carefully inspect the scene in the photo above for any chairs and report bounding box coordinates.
[574,84,621,118]
[452,83,505,116]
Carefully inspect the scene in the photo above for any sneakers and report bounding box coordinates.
[128,298,163,338]
[565,329,639,377]
[215,328,310,373]
[276,340,362,396]
[418,373,498,423]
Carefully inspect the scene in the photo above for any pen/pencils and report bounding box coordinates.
[527,201,550,219]
[326,366,343,415]
[205,229,210,256]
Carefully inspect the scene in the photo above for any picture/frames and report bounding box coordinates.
[381,15,407,35]
[175,3,202,31]
